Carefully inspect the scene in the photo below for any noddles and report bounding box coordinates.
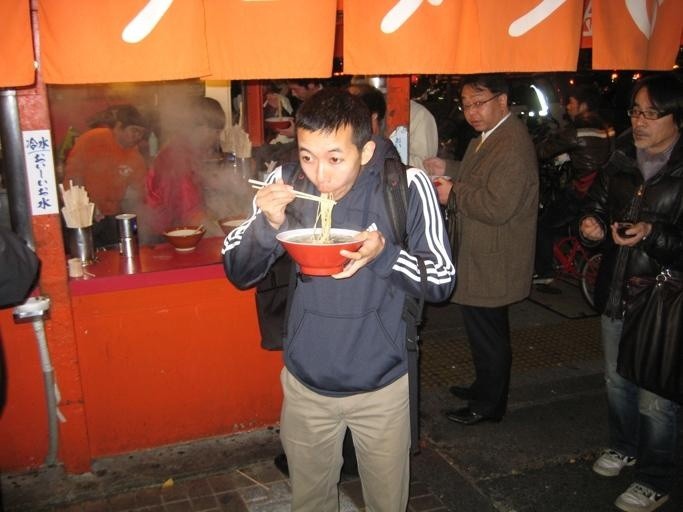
[302,192,349,244]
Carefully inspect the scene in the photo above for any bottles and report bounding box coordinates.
[115,214,139,257]
[118,256,142,276]
[148,132,157,157]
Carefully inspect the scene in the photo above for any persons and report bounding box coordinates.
[1,230,40,412]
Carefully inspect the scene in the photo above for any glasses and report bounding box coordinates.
[626,108,673,121]
[457,91,503,112]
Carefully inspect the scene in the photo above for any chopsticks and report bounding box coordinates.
[192,224,202,236]
[247,179,337,206]
[58,179,95,228]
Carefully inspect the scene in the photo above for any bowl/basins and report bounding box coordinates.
[218,214,252,237]
[276,227,365,277]
[427,176,451,187]
[160,226,206,251]
[265,116,294,132]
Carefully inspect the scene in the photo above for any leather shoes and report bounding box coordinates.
[448,385,473,400]
[445,405,503,426]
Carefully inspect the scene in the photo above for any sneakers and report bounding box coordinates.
[532,272,557,285]
[591,444,638,478]
[613,481,672,512]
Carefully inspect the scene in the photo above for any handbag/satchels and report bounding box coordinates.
[442,188,458,262]
[614,274,683,407]
[253,253,298,354]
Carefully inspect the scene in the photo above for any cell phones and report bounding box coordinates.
[615,220,635,238]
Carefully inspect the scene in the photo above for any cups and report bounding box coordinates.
[63,225,93,267]
